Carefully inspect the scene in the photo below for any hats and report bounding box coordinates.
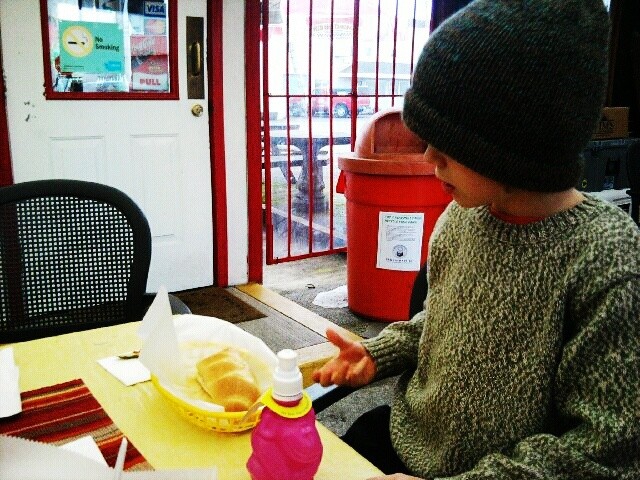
[403,1,614,190]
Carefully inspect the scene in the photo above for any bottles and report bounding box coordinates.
[244,348,325,480]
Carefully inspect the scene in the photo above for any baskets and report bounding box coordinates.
[149,341,272,434]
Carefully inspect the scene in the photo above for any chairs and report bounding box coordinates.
[0,178,192,346]
[302,256,428,416]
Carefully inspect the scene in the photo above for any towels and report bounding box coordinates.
[0,376,155,472]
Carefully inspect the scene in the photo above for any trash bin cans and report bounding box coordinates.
[336,107,453,322]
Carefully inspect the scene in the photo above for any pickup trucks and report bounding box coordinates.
[289,86,370,118]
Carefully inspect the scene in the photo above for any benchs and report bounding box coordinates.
[263,153,330,182]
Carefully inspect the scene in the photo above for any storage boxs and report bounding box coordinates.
[590,106,630,140]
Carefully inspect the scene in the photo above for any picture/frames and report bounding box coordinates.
[39,0,180,101]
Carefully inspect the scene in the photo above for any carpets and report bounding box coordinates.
[169,285,268,324]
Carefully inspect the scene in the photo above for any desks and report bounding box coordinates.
[262,127,358,213]
[261,119,301,184]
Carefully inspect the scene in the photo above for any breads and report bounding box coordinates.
[196,345,259,412]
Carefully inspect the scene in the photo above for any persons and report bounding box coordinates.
[312,0,640,480]
